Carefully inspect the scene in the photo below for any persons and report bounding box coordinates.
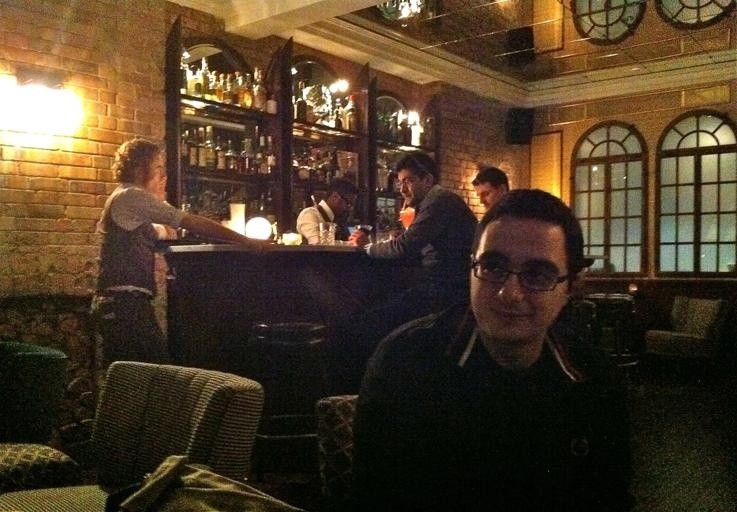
[470,167,510,213]
[297,177,358,246]
[91,138,278,367]
[352,188,635,512]
[346,150,479,322]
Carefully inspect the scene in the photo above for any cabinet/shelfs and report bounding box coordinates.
[287,55,368,244]
[175,35,285,241]
[370,90,437,247]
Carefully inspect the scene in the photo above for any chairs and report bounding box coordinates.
[643,293,728,385]
[316,394,368,512]
[1,341,68,442]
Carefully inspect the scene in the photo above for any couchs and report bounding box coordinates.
[1,358,265,512]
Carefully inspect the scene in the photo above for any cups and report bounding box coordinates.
[398,207,415,230]
[356,224,372,236]
[283,233,302,245]
[319,222,337,244]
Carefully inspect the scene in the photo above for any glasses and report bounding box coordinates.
[471,260,572,292]
[395,179,415,188]
[343,197,353,209]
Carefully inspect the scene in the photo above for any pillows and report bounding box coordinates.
[0,442,82,489]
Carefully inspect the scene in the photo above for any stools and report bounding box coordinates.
[254,318,330,490]
[581,291,641,371]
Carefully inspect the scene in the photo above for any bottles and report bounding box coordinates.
[255,186,277,226]
[292,80,315,123]
[179,54,279,113]
[182,125,277,180]
[378,159,393,193]
[332,94,357,130]
